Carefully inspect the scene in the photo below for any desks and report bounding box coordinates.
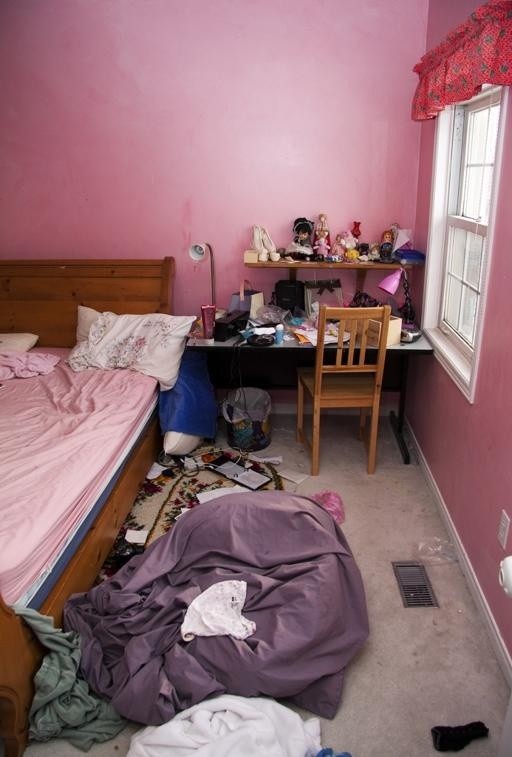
[186,310,434,464]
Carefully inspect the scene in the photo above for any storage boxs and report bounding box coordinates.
[368,316,403,347]
[395,249,426,265]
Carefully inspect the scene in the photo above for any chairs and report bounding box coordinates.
[296,303,393,476]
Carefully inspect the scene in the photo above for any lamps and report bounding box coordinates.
[188,241,227,320]
[377,266,416,329]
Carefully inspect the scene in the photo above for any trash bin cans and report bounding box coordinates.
[222,387,273,453]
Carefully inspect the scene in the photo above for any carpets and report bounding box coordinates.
[91,443,284,590]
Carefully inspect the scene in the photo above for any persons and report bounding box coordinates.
[285,217,315,262]
[378,230,395,264]
[314,230,332,261]
[331,234,343,259]
[317,212,329,231]
[342,230,359,251]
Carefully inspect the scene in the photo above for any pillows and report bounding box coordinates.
[0,332,40,353]
[158,352,221,456]
[65,306,198,391]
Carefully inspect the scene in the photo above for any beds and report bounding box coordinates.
[1,255,178,757]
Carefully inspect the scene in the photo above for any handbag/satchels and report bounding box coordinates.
[229,278,265,320]
[304,269,344,318]
